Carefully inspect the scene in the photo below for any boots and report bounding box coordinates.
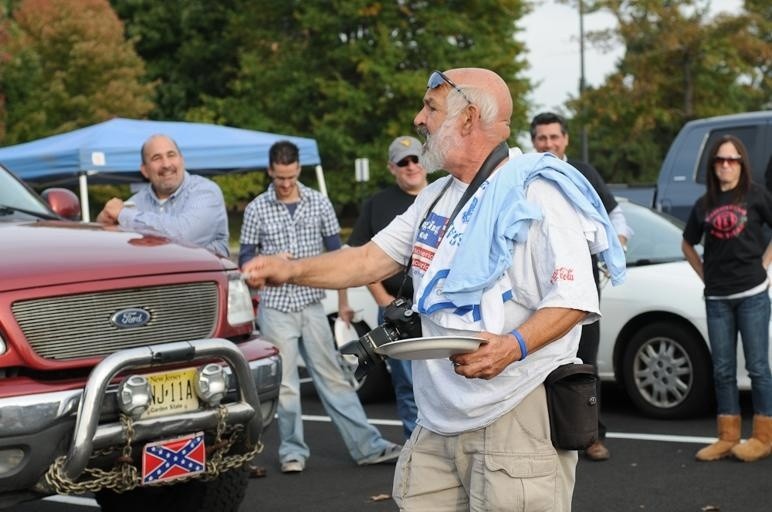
[730,415,771,462]
[696,415,741,462]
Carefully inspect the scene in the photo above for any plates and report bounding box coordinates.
[375,337,486,357]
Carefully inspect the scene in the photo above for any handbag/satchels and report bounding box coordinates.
[542,360,601,450]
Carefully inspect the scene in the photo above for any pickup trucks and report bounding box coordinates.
[611,112,772,238]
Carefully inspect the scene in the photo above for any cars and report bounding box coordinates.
[289,183,767,429]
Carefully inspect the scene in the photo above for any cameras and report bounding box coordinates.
[339,301,421,386]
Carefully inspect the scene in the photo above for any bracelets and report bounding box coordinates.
[510,330,529,361]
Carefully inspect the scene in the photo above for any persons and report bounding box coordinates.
[679,136,772,461]
[242,65,606,511]
[528,112,630,463]
[94,131,232,261]
[350,136,433,445]
[240,139,405,475]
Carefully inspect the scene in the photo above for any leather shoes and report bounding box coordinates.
[234,459,267,479]
[582,441,610,461]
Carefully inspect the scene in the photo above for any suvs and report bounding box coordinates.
[0,154,287,512]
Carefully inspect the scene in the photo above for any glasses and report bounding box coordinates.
[397,156,419,167]
[427,70,481,119]
[714,156,742,166]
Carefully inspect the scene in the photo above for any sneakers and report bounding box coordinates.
[358,443,404,466]
[281,459,303,472]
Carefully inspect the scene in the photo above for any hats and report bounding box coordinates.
[389,135,423,164]
